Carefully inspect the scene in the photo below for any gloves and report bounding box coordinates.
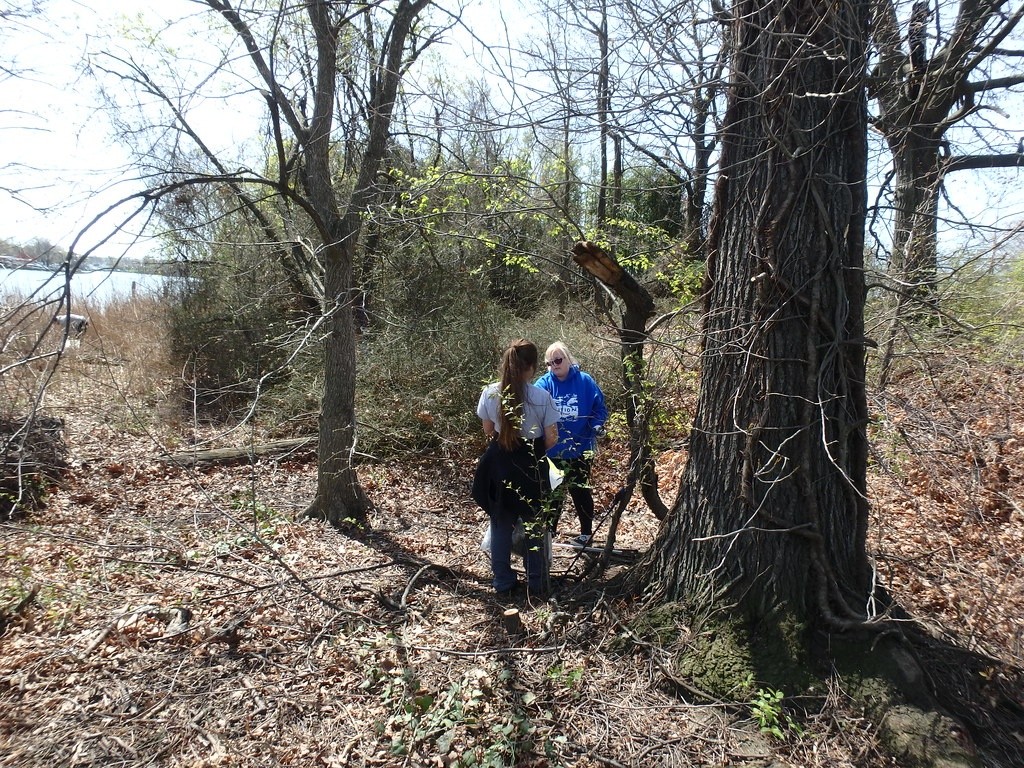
[592,425,605,438]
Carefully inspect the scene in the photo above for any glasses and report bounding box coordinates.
[547,356,570,366]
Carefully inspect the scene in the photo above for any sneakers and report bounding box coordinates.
[574,535,593,546]
[552,533,556,538]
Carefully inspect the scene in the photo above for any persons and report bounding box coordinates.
[476,338,559,600]
[53,313,89,346]
[531,341,608,548]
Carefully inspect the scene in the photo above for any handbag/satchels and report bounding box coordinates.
[481,516,525,557]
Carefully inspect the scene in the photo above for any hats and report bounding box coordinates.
[547,456,566,493]
